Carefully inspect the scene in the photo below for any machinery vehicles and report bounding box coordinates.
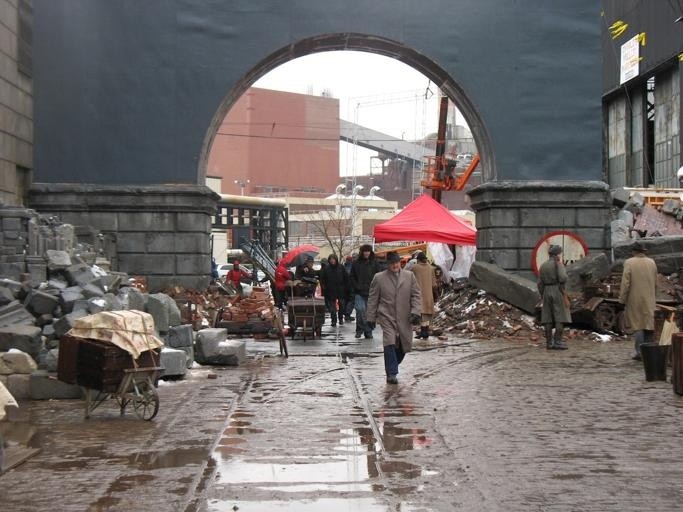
[361,85,482,284]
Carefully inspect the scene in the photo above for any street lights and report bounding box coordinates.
[233,176,251,198]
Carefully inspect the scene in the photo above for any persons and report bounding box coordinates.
[537,244,572,350]
[365,250,423,385]
[260,245,437,341]
[618,241,658,361]
[225,261,256,290]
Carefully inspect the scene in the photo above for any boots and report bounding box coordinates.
[415,326,428,340]
[546,328,568,349]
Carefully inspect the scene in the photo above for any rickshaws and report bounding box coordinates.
[210,302,294,360]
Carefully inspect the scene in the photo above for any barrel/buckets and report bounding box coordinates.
[639,342,670,383]
[227,248,243,260]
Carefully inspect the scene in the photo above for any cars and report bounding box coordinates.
[215,262,249,281]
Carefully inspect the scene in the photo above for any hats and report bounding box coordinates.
[549,245,562,255]
[380,251,402,263]
[630,242,648,251]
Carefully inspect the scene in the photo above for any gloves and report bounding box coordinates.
[408,315,420,324]
[367,321,376,330]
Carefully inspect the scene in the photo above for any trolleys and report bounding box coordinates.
[79,336,164,421]
[284,280,329,341]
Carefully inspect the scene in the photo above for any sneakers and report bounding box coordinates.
[387,374,398,383]
